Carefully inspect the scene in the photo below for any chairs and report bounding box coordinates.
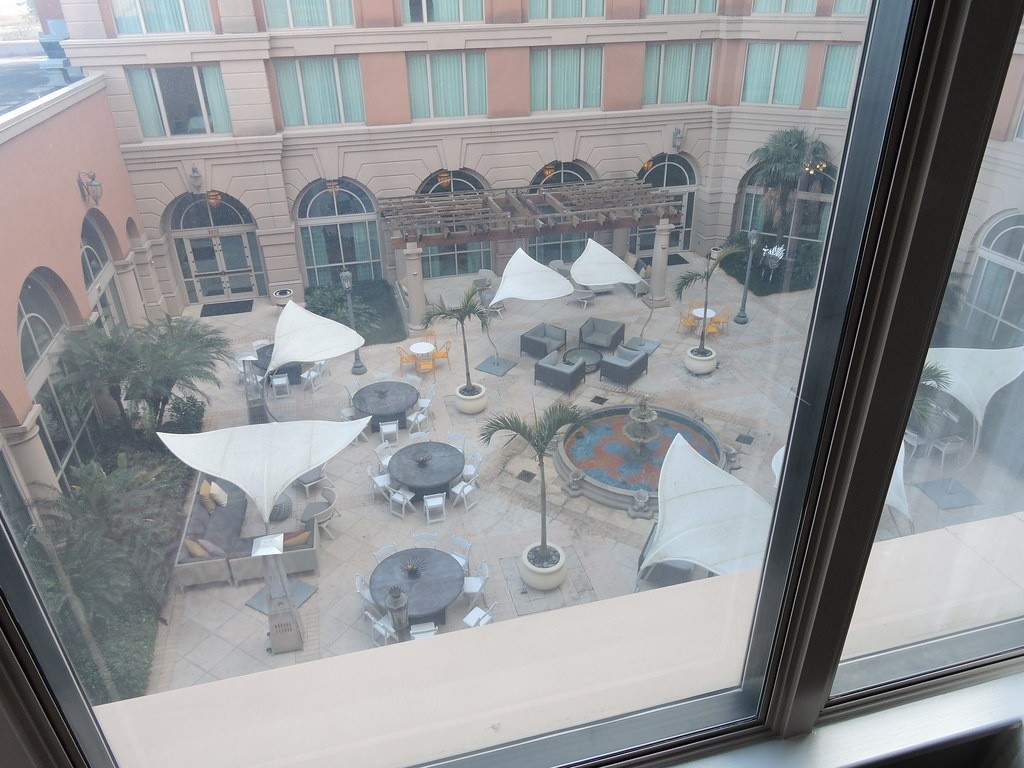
[710,307,732,335]
[637,522,697,585]
[600,344,648,391]
[705,319,720,342]
[578,317,625,356]
[520,321,567,359]
[902,429,929,462]
[340,329,481,525]
[549,259,595,311]
[677,310,696,335]
[291,458,336,498]
[232,335,332,400]
[926,435,969,471]
[473,268,494,288]
[478,289,507,320]
[354,531,498,646]
[300,487,342,540]
[534,350,586,397]
[687,300,704,325]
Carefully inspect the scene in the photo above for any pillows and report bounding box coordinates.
[626,255,638,269]
[405,295,409,303]
[197,538,226,556]
[209,481,228,508]
[400,277,408,286]
[199,480,216,515]
[402,285,408,294]
[183,539,209,557]
[284,530,303,540]
[646,265,652,279]
[624,251,637,263]
[283,532,311,547]
[639,267,646,279]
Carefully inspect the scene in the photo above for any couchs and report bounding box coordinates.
[174,470,247,595]
[619,257,652,297]
[228,517,323,589]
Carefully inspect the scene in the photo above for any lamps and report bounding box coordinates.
[543,164,557,180]
[672,127,683,153]
[437,172,451,189]
[804,160,826,175]
[326,181,340,197]
[77,170,104,206]
[189,164,202,192]
[206,191,221,208]
[643,158,654,172]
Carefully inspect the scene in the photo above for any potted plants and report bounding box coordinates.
[420,285,497,415]
[480,394,599,590]
[674,240,749,374]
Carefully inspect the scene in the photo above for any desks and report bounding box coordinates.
[563,347,602,374]
[587,285,616,293]
[269,493,292,521]
[693,308,716,336]
[353,381,419,434]
[389,441,465,506]
[251,343,302,387]
[368,547,466,625]
[440,290,469,309]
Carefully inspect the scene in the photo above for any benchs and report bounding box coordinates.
[396,281,435,323]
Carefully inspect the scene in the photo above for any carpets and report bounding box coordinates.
[200,299,253,317]
[640,253,690,267]
